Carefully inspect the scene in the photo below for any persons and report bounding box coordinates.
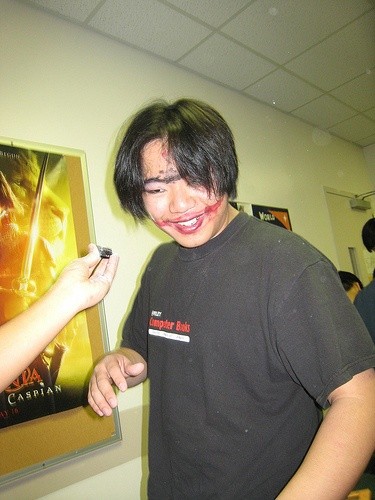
[89,99,375,500]
[0,244,120,395]
